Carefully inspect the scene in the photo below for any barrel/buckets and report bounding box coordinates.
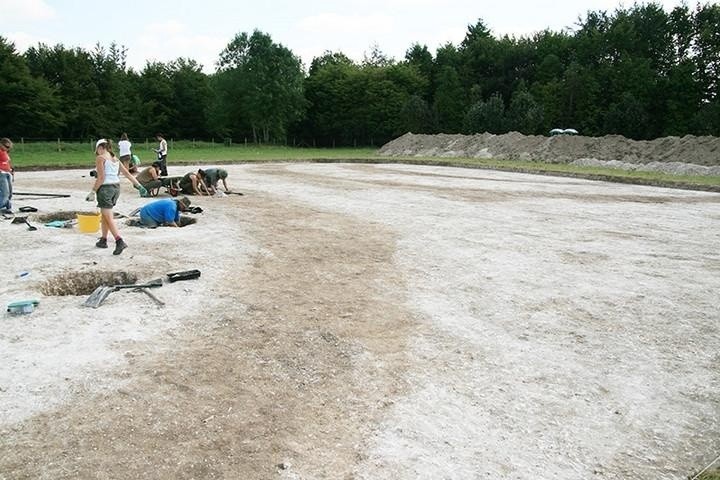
[76,213,101,233]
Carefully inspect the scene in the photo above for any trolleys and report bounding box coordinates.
[152,175,185,197]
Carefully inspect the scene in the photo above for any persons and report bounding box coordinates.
[124,197,193,229]
[154,133,170,177]
[129,153,141,174]
[118,132,132,178]
[201,165,232,194]
[84,138,148,255]
[0,138,16,215]
[132,161,162,199]
[178,167,212,197]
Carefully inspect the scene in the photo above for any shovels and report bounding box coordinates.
[12,217,37,231]
[82,284,162,308]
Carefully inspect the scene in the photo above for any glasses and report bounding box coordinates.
[3,144,10,148]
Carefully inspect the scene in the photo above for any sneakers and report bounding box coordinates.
[6,209,14,213]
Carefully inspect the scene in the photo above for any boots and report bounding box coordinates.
[96,237,108,248]
[113,238,128,254]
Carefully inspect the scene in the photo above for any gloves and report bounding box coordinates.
[136,184,147,194]
[86,191,95,201]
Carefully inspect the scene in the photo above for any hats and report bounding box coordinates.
[153,161,163,170]
[200,170,205,180]
[179,196,191,208]
[95,139,108,155]
[219,169,227,179]
